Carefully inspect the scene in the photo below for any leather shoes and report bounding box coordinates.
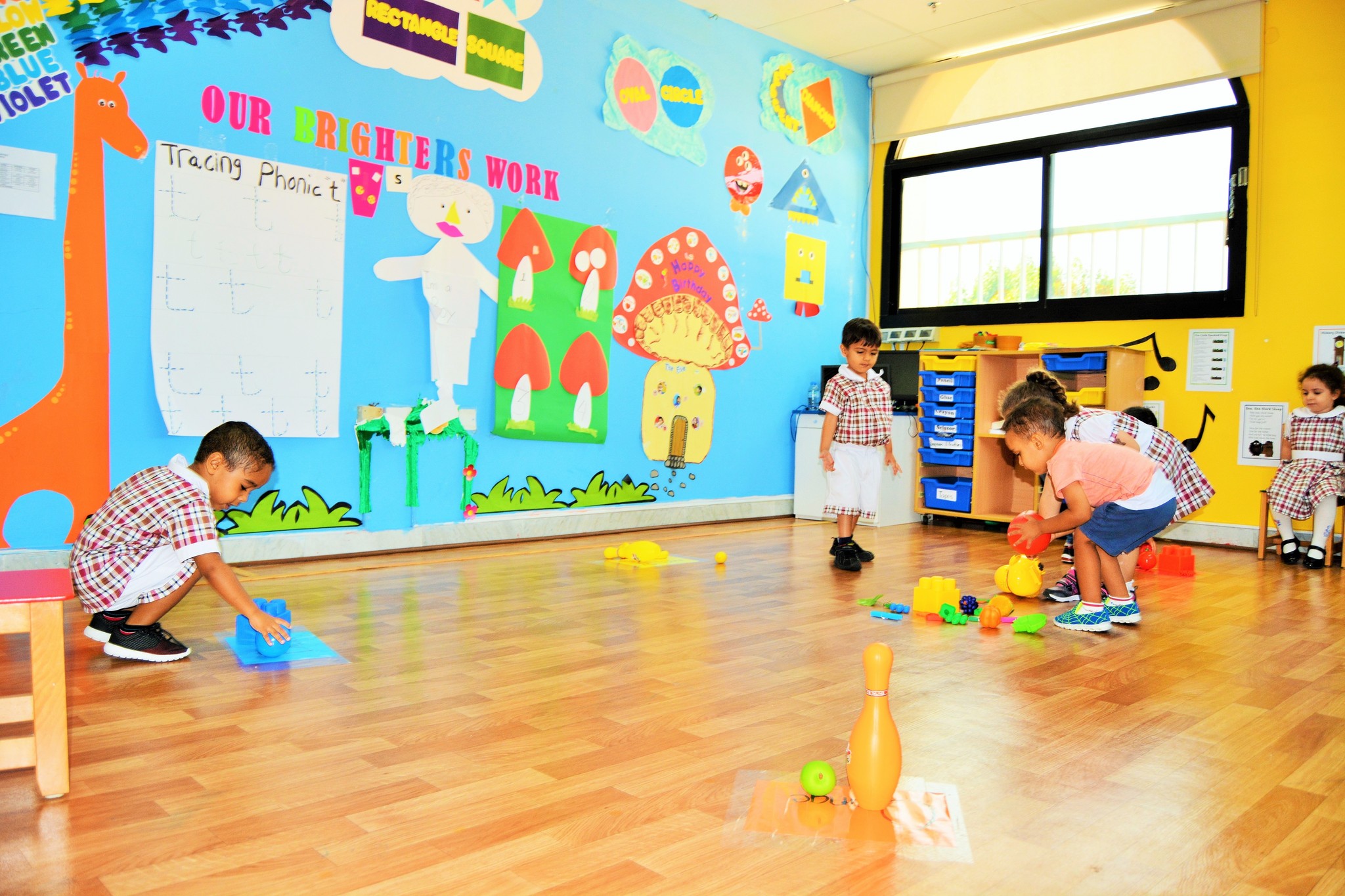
[1280,534,1301,565]
[1303,545,1327,569]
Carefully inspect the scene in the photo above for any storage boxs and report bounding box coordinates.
[1039,352,1106,373]
[916,368,978,467]
[1063,384,1108,408]
[918,474,974,515]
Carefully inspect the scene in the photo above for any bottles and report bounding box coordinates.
[807,382,819,410]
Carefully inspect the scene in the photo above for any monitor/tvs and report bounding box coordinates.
[819,364,890,408]
[871,348,920,407]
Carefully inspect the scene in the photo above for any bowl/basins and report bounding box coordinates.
[973,336,995,348]
[996,335,1022,351]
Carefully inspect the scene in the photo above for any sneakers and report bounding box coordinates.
[83,610,132,642]
[1060,543,1074,562]
[834,542,862,571]
[1043,568,1082,601]
[1102,596,1141,623]
[104,621,192,662]
[1099,582,1138,602]
[1053,600,1112,632]
[830,537,875,562]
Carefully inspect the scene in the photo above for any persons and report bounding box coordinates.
[1061,407,1157,552]
[70,421,292,663]
[998,369,1215,607]
[1267,363,1345,569]
[1000,395,1177,632]
[818,317,902,572]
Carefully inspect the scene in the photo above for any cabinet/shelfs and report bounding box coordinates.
[910,344,1149,521]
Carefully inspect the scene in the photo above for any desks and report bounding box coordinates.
[786,409,934,529]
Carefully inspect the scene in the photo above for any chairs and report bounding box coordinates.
[0,568,76,799]
[1255,487,1345,571]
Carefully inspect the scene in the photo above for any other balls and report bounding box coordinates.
[1138,551,1158,569]
[715,551,728,564]
[1007,510,1053,554]
[256,622,291,656]
